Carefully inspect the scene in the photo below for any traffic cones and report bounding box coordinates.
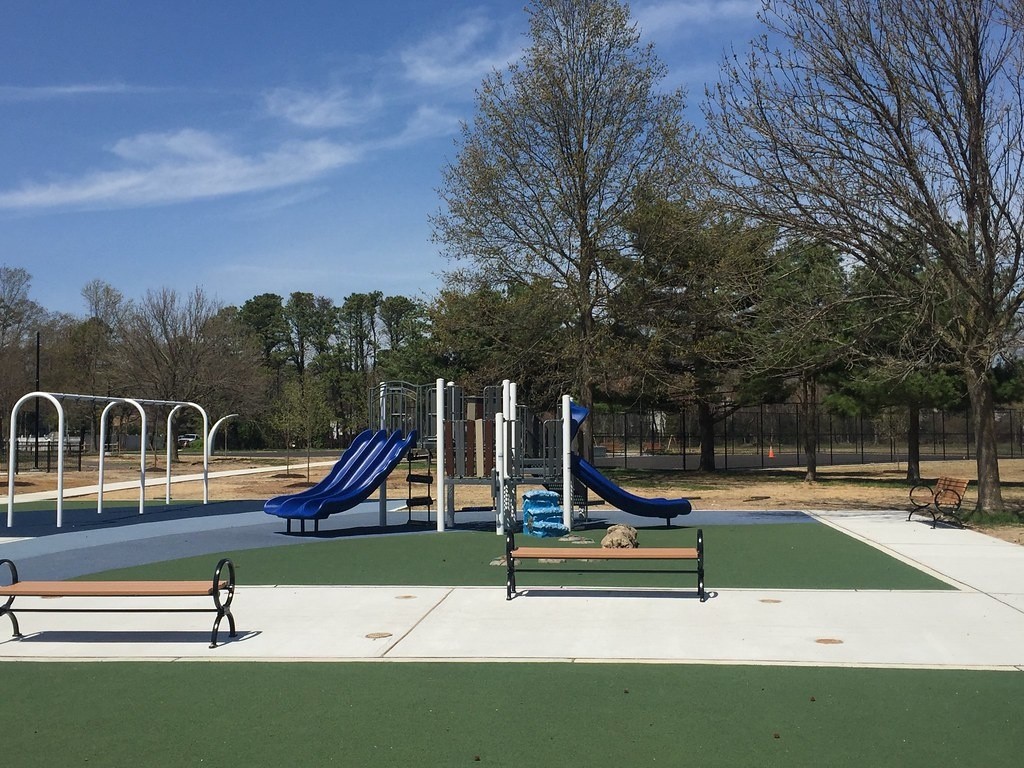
[768,446,775,458]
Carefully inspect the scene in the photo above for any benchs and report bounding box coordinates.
[905,476,970,530]
[505,528,706,602]
[0,558,238,649]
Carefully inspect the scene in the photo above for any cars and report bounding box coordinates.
[178,434,202,447]
[44,432,59,440]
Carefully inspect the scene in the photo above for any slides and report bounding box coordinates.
[571,452,692,518]
[264,428,418,520]
